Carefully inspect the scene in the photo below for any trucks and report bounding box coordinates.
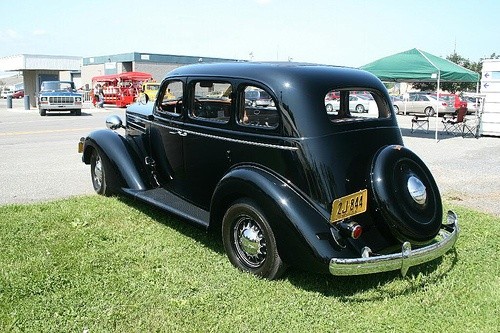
[13,91,24,98]
[91,72,175,108]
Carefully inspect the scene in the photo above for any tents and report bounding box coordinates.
[355,47,479,142]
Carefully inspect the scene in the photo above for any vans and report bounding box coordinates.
[206,91,225,98]
[245,91,270,107]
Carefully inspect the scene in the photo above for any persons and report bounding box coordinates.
[94,84,104,108]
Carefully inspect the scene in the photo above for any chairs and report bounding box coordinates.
[440,107,474,138]
[411,113,430,134]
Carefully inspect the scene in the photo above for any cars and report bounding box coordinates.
[392,91,480,117]
[325,93,402,113]
[78,62,458,283]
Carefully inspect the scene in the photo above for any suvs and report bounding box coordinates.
[38,81,82,116]
